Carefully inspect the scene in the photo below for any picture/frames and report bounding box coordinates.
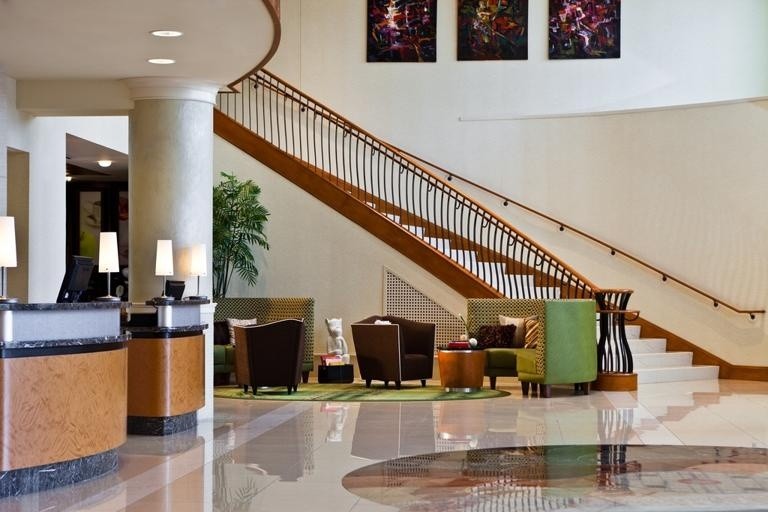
[548,0,621,60]
[366,0,437,63]
[457,0,528,61]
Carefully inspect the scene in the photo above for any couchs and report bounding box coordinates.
[467,297,598,397]
[213,298,314,384]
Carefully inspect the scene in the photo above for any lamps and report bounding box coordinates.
[155,239,175,301]
[99,232,121,302]
[0,216,18,301]
[188,244,208,300]
[97,158,113,168]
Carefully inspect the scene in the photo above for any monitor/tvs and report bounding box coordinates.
[162,280,185,300]
[56,256,94,303]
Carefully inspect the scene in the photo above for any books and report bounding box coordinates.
[320,354,345,366]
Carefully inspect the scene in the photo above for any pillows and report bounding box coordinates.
[214,321,231,345]
[374,319,392,326]
[498,314,538,347]
[224,317,258,346]
[473,324,516,350]
[524,319,538,349]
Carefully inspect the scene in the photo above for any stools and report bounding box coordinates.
[318,364,354,384]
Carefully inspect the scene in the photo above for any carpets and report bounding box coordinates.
[214,384,511,401]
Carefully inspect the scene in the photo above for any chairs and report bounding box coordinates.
[232,319,305,396]
[351,315,435,390]
